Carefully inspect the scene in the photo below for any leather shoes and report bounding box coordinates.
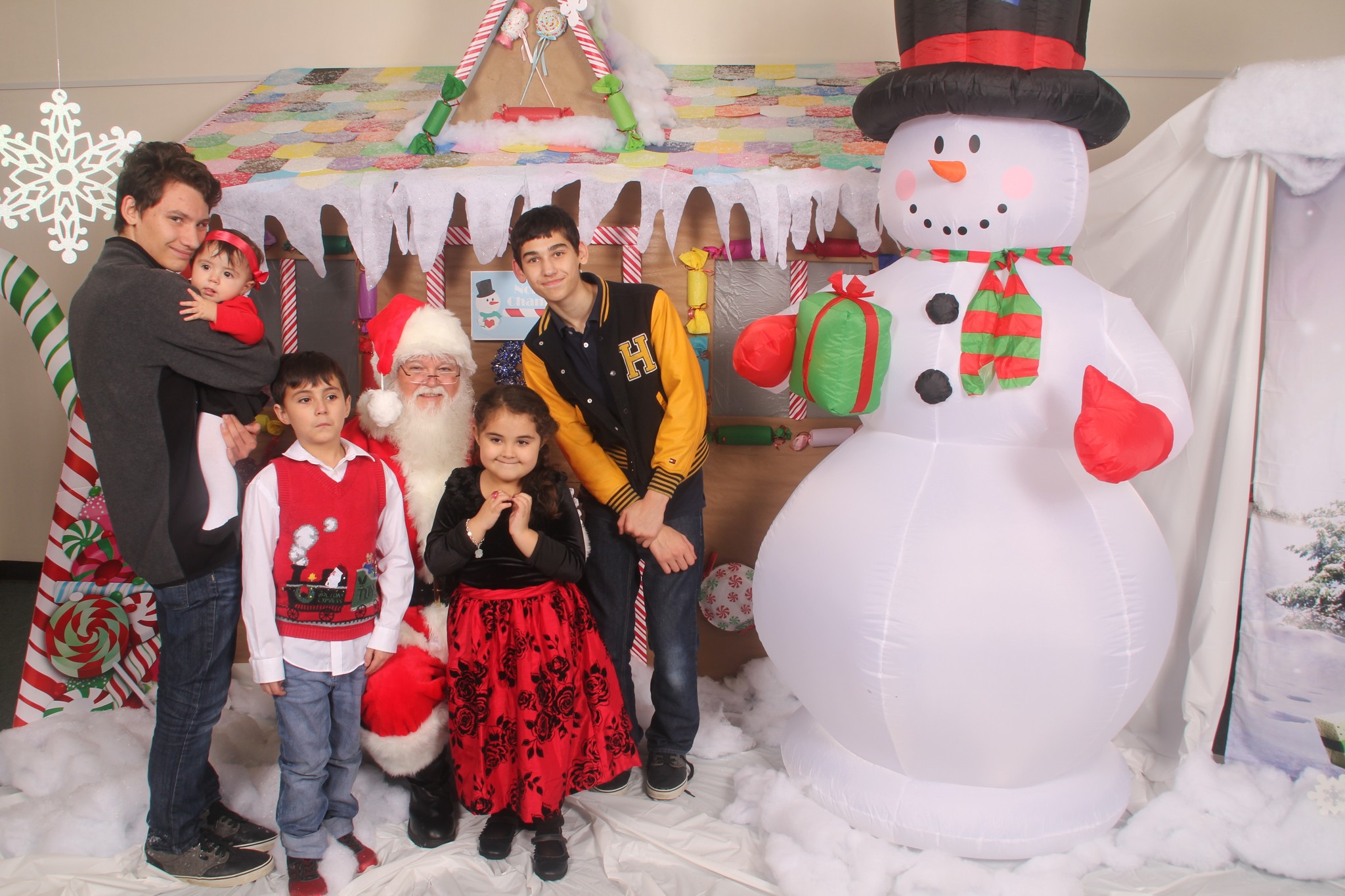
[479,816,522,860]
[531,827,569,881]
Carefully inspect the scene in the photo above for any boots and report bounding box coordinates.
[405,744,455,849]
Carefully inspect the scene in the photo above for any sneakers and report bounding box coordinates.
[646,752,695,800]
[145,827,275,887]
[336,831,380,875]
[286,855,328,896]
[202,801,280,852]
[590,767,632,796]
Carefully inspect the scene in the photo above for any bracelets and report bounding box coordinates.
[466,518,485,558]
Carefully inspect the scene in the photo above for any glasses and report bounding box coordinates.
[399,365,460,385]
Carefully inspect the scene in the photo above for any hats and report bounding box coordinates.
[366,293,477,427]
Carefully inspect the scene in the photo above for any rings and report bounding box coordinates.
[491,491,499,499]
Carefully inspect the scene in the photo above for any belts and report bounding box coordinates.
[409,574,449,608]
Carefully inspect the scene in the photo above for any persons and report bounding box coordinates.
[424,384,642,882]
[69,142,281,886]
[242,351,416,896]
[340,293,483,850]
[176,230,270,548]
[512,206,709,800]
